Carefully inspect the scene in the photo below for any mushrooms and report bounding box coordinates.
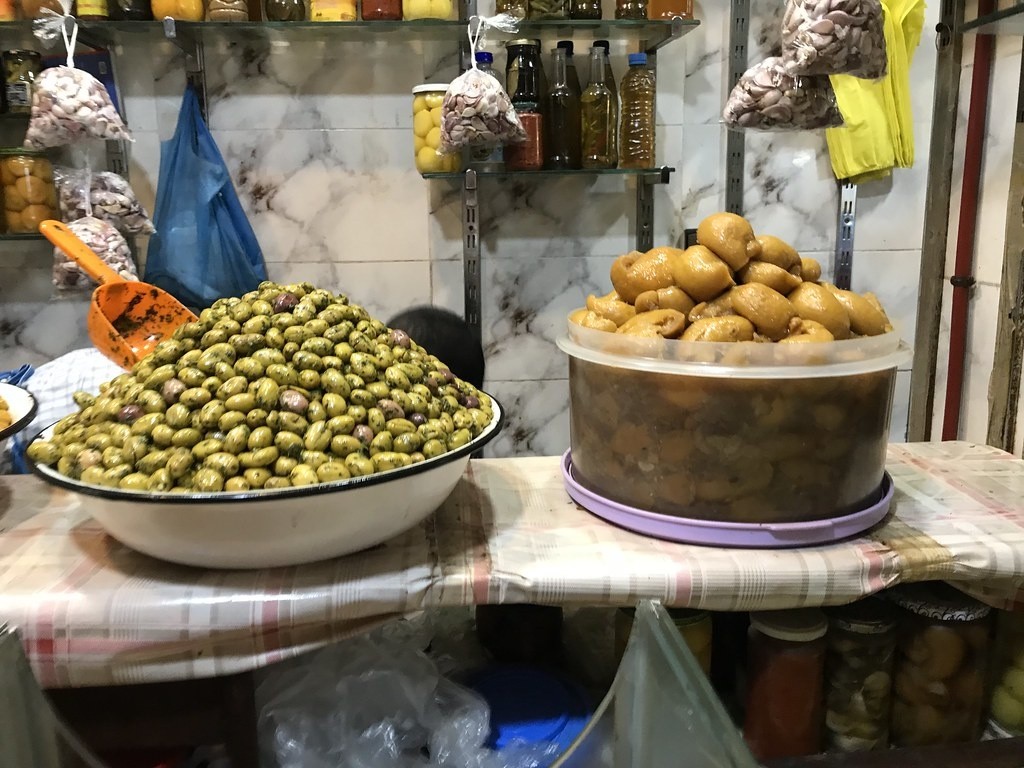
[722,0,888,132]
[23,67,155,297]
[441,73,526,156]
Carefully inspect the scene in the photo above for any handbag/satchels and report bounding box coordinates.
[139,91,268,312]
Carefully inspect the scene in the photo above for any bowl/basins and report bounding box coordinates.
[0,382,36,456]
[27,391,505,571]
[553,307,914,524]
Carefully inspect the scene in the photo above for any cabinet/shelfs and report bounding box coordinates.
[0,443,1024,768]
[0,16,699,242]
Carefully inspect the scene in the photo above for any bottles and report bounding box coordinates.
[471,52,505,173]
[618,53,656,168]
[648,1,694,21]
[581,47,616,169]
[506,37,546,170]
[557,41,581,102]
[593,40,617,97]
[546,47,579,169]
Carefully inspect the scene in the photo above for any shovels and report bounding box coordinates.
[39,218,201,372]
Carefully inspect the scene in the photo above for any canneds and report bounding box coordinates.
[503,101,543,172]
[0,146,60,234]
[0,48,41,116]
[412,82,465,174]
[667,588,1024,761]
